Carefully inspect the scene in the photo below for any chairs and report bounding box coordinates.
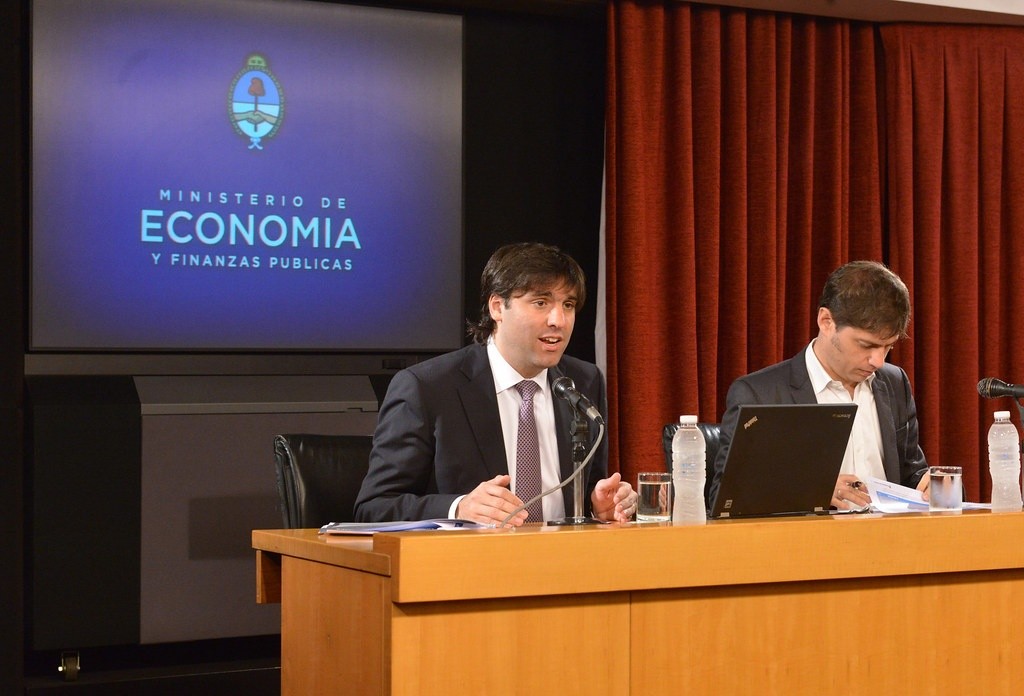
[659,422,722,512]
[273,431,374,527]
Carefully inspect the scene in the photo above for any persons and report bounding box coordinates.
[709,261,951,510]
[353,242,639,531]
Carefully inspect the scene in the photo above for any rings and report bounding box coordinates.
[837,488,843,500]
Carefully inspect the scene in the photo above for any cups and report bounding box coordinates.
[638,473,672,526]
[928,465,964,515]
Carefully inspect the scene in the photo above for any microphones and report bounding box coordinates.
[977,377,1024,399]
[552,377,604,426]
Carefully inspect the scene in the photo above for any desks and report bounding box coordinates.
[252,503,1024,696]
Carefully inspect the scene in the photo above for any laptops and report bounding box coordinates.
[713,402,859,518]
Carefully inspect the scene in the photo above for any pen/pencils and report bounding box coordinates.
[848,480,864,488]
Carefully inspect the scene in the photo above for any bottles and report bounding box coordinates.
[987,410,1024,514]
[670,415,707,528]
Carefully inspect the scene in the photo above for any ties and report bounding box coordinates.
[515,380,543,523]
